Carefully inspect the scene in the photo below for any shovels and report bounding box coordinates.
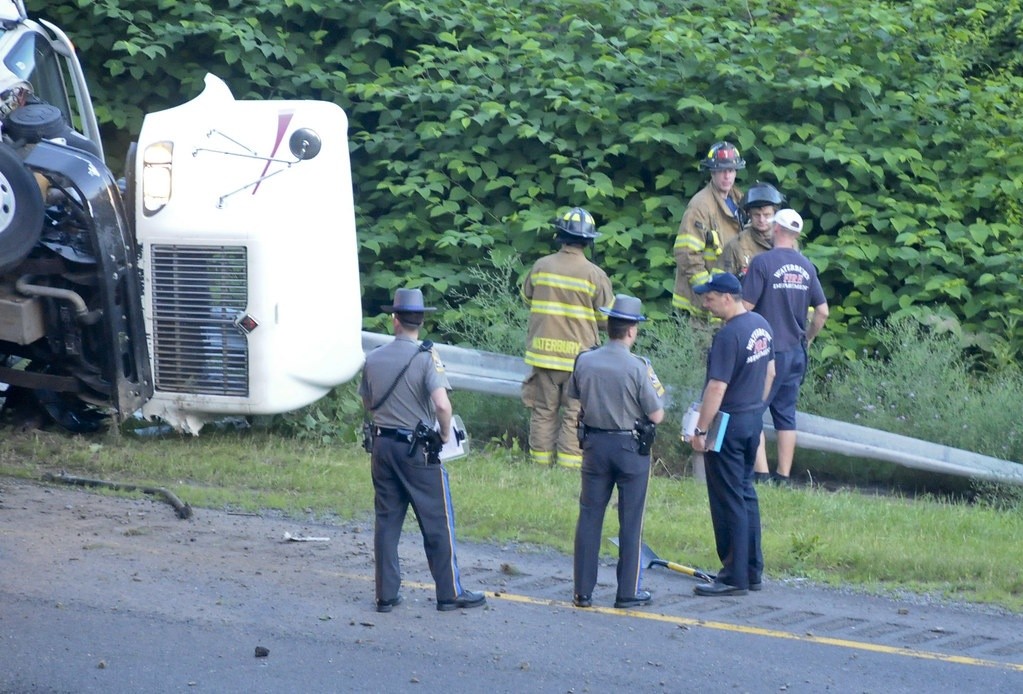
[608,535,716,582]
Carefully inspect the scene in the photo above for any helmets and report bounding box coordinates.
[547,205,602,238]
[698,141,746,171]
[738,180,783,208]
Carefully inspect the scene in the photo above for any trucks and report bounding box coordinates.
[0,1,365,445]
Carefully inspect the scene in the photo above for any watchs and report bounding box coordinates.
[694,427,706,436]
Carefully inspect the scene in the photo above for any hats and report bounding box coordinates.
[598,293,646,322]
[692,272,742,295]
[767,208,804,233]
[380,288,438,312]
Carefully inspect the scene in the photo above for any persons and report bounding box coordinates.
[567,294,665,608]
[679,271,776,597]
[671,141,745,331]
[718,182,785,279]
[520,207,616,470]
[359,287,487,612]
[737,208,829,487]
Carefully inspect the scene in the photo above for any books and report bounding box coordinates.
[678,400,730,453]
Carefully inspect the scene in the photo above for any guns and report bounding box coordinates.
[634,418,652,436]
[407,419,430,456]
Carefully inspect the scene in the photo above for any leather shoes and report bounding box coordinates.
[613,590,651,607]
[749,581,762,591]
[692,578,749,597]
[377,593,404,612]
[437,589,487,611]
[572,592,593,607]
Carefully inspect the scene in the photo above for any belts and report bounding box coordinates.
[584,425,634,436]
[373,427,413,443]
